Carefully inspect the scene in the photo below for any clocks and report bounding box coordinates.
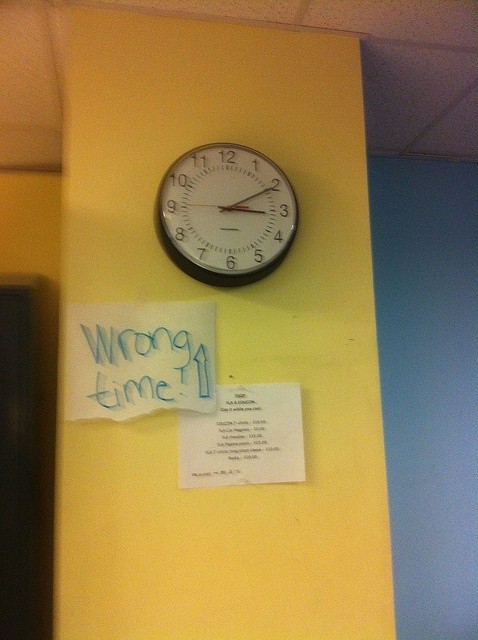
[153,143,300,288]
